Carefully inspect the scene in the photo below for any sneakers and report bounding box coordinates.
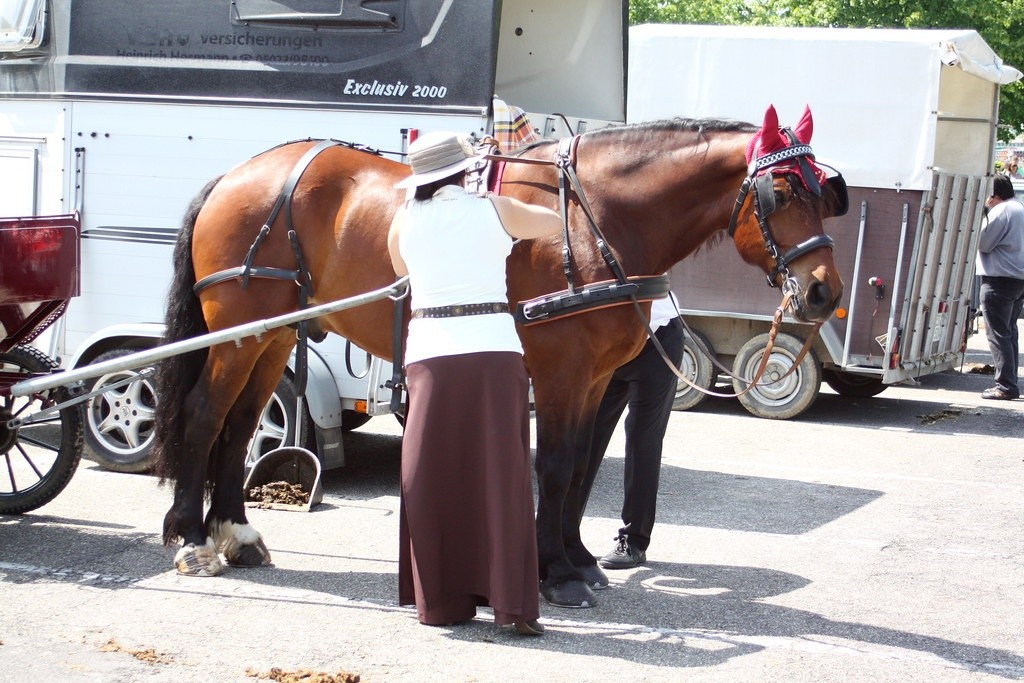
[599,533,646,569]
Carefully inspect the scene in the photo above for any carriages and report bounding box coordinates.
[1,101,851,614]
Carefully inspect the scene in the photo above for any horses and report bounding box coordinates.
[157,101,841,607]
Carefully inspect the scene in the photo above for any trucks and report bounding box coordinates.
[2,0,634,486]
[625,22,1021,421]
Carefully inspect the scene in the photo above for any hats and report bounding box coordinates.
[392,132,488,190]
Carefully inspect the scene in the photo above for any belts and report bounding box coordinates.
[409,303,511,319]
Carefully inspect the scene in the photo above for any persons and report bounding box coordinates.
[999,156,1024,179]
[975,174,1024,400]
[386,128,564,635]
[569,291,685,570]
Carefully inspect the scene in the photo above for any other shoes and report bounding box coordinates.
[514,620,545,635]
[981,386,1020,400]
